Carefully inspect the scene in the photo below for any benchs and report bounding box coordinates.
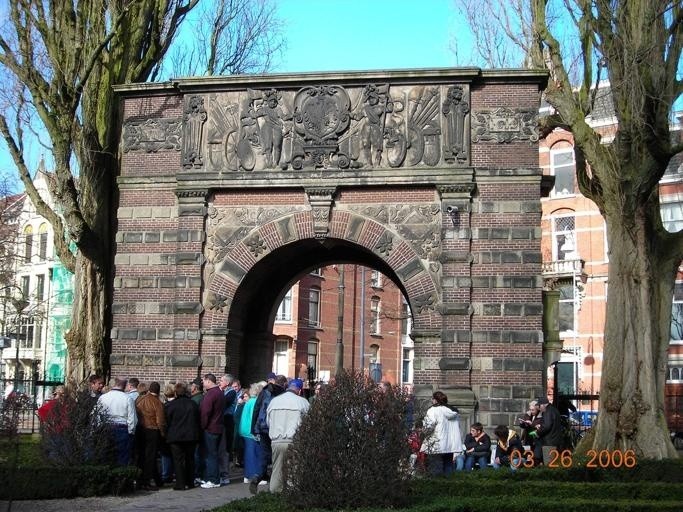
[473,443,531,470]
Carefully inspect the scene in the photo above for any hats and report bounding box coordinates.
[288,378,303,390]
[535,397,549,407]
[266,372,276,381]
[189,376,203,391]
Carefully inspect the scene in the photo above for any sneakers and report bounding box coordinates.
[243,476,253,484]
[257,479,268,486]
[137,475,230,492]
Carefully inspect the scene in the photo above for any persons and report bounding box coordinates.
[363,381,576,480]
[38,372,337,495]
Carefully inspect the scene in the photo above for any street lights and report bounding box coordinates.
[11,299,31,392]
[558,224,579,414]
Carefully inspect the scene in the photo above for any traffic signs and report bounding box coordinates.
[7,333,27,340]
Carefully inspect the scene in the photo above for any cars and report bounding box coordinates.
[569,411,600,432]
